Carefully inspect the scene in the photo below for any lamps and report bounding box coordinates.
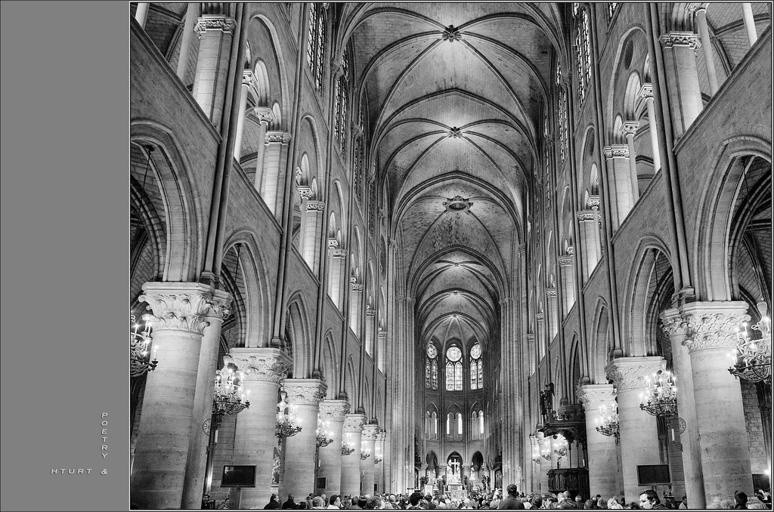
[130,319,159,378]
[214,364,249,416]
[596,414,620,440]
[640,369,677,418]
[315,428,333,447]
[728,321,773,382]
[275,413,301,439]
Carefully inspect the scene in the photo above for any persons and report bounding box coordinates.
[264,485,688,509]
[201,494,210,510]
[733,490,748,509]
[218,498,230,509]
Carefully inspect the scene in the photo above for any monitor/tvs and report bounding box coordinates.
[317,477,327,489]
[220,465,256,488]
[374,484,377,491]
[637,464,672,486]
